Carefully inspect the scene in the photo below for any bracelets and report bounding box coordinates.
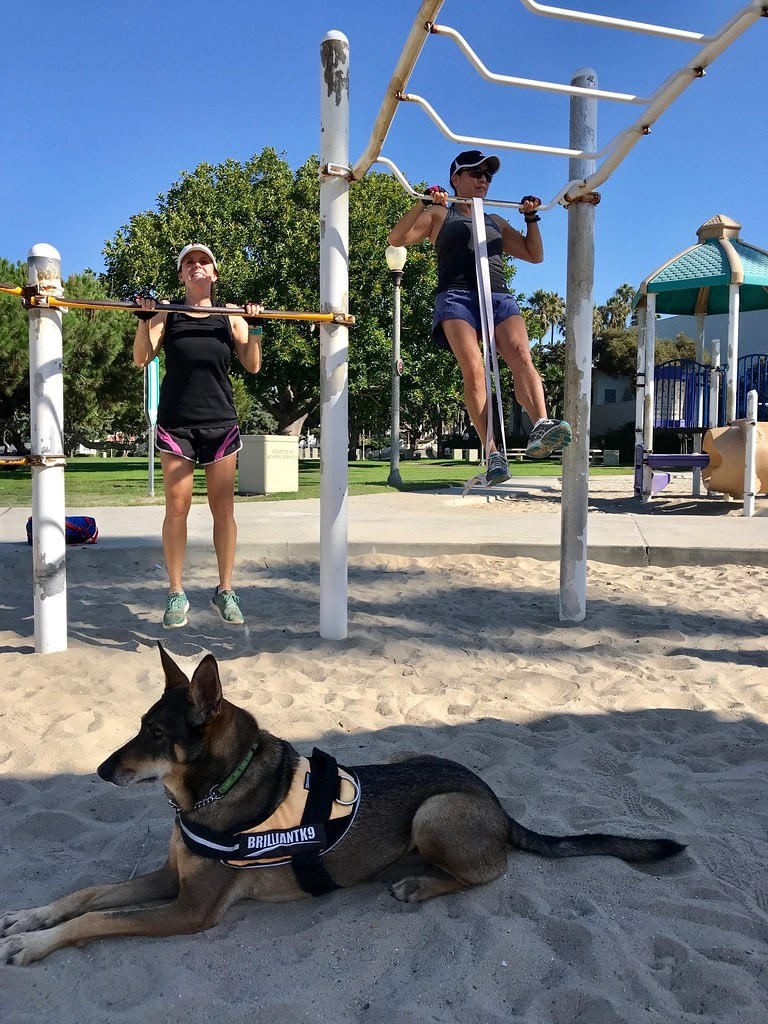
[525,215,541,223]
[248,326,262,335]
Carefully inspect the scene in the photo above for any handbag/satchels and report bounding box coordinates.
[26,515,99,545]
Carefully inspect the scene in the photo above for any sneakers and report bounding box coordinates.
[163,592,189,629]
[484,451,511,487]
[526,418,571,459]
[210,584,244,624]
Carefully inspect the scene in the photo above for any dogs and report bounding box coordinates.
[0,639,688,969]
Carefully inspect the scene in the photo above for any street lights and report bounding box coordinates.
[385,243,408,494]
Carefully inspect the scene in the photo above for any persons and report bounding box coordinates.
[133,243,264,629]
[389,150,572,488]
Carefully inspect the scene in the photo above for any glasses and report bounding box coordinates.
[459,167,493,183]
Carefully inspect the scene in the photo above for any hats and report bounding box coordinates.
[177,243,217,271]
[450,150,501,182]
